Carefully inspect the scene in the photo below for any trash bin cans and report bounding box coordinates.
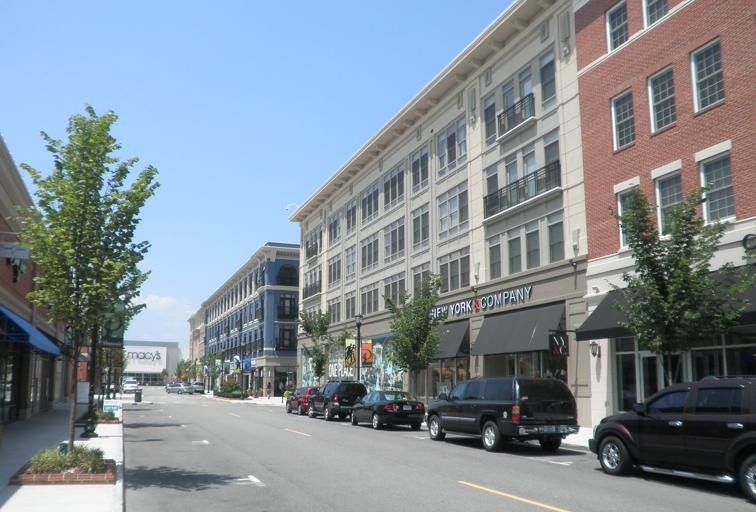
[213,390,217,394]
[135,389,142,402]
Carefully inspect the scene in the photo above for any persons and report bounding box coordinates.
[274,380,296,397]
[266,382,272,399]
[247,382,263,399]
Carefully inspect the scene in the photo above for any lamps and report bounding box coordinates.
[589,341,601,359]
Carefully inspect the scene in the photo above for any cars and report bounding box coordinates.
[192,381,205,394]
[165,380,194,395]
[285,386,318,415]
[349,390,425,430]
[122,379,140,393]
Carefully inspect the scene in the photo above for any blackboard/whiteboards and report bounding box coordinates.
[76,382,89,403]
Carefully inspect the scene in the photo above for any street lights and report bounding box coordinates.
[353,313,364,382]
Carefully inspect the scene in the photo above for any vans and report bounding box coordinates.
[424,376,581,452]
[588,373,756,502]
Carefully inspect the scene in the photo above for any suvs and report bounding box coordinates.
[307,379,367,421]
